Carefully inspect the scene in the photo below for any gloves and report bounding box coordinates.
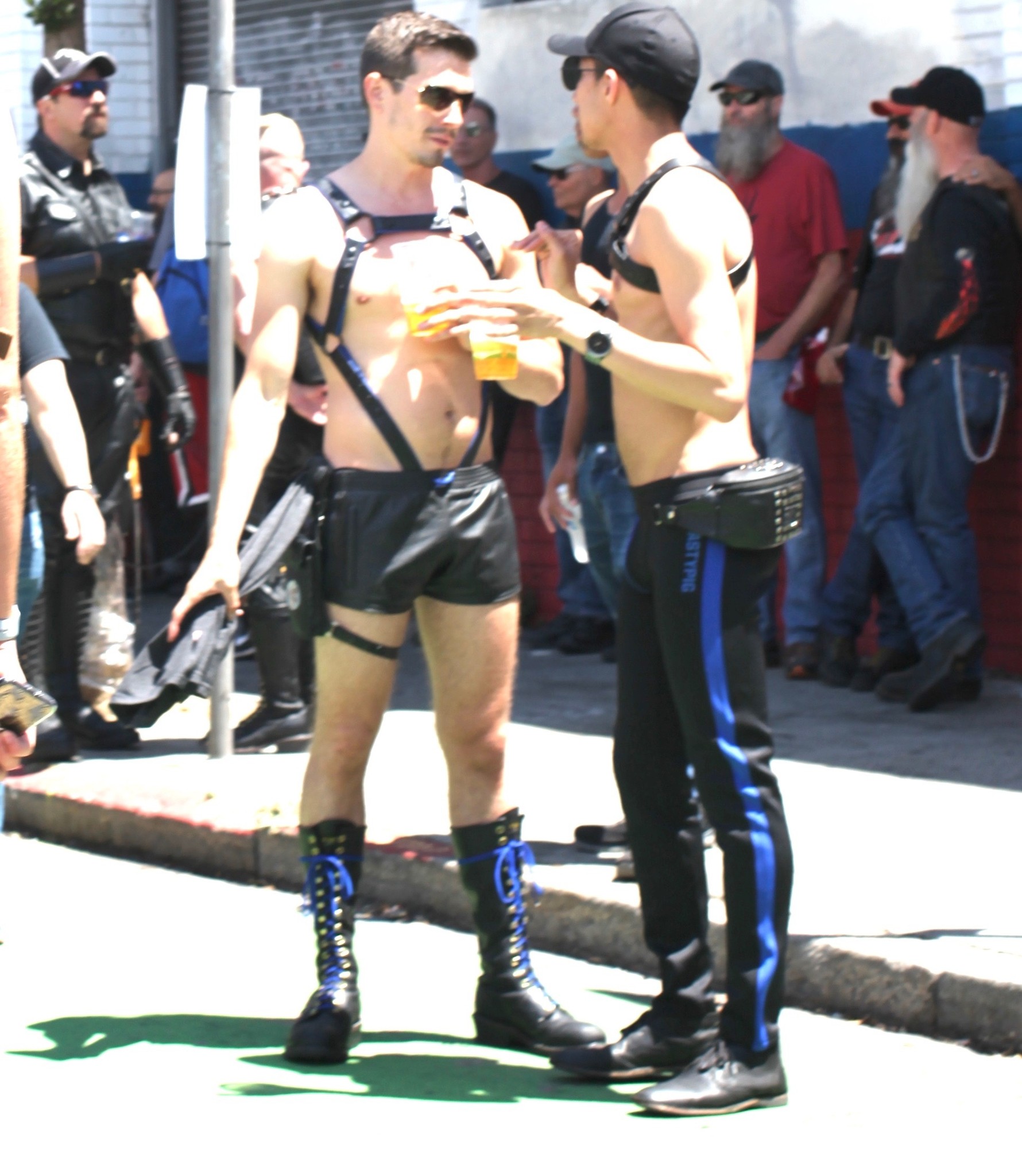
[138,393,196,453]
[94,219,155,286]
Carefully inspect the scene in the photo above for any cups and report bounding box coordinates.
[390,237,462,336]
[470,283,519,380]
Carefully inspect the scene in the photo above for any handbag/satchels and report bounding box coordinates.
[665,456,806,550]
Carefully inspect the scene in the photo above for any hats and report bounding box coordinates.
[869,81,920,115]
[547,1,701,103]
[710,61,784,95]
[530,141,617,176]
[31,47,117,105]
[891,67,984,127]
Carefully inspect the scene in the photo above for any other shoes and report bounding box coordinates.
[524,613,616,654]
[763,616,986,712]
[574,804,717,881]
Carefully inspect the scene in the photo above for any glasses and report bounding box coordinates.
[380,74,474,115]
[886,118,911,129]
[49,79,107,99]
[557,163,587,180]
[561,57,607,91]
[462,122,492,137]
[719,90,769,106]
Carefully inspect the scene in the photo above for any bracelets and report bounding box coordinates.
[64,482,102,501]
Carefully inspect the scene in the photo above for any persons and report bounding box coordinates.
[415,1,792,1118]
[0,47,1022,772]
[170,12,608,1065]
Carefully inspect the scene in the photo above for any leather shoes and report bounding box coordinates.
[550,1007,721,1079]
[632,1038,788,1116]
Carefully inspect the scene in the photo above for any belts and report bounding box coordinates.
[847,330,895,359]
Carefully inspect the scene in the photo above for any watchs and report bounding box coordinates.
[585,329,613,365]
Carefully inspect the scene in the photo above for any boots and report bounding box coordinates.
[234,603,315,751]
[23,549,142,764]
[283,818,367,1063]
[448,805,606,1056]
